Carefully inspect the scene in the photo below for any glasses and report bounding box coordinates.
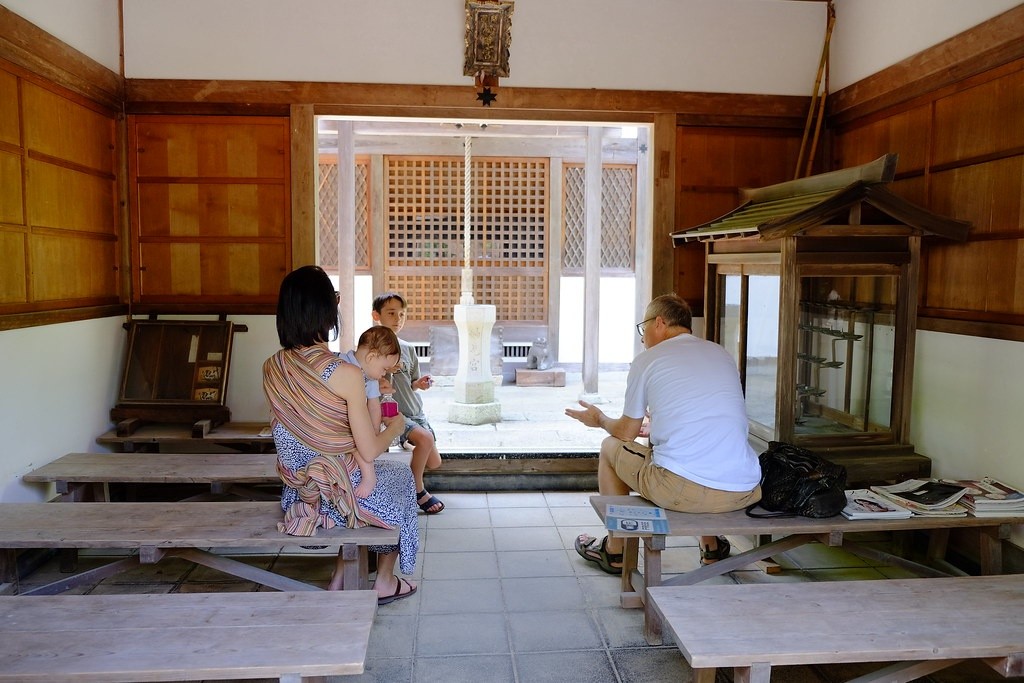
[335,291,340,304]
[636,317,665,336]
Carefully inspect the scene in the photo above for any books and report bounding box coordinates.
[836,477,1024,521]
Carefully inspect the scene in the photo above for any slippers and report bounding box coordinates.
[378,575,417,604]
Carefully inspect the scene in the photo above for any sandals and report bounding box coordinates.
[699,535,730,567]
[417,489,444,514]
[574,535,624,575]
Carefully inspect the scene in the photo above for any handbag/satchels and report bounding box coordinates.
[745,441,847,518]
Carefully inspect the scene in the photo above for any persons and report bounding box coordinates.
[563,294,764,574]
[854,499,898,513]
[261,264,423,607]
[370,293,446,515]
[332,324,402,501]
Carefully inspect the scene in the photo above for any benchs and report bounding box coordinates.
[0,589,380,683]
[96,421,277,453]
[0,502,401,592]
[22,453,414,502]
[644,574,1024,683]
[589,479,1024,646]
